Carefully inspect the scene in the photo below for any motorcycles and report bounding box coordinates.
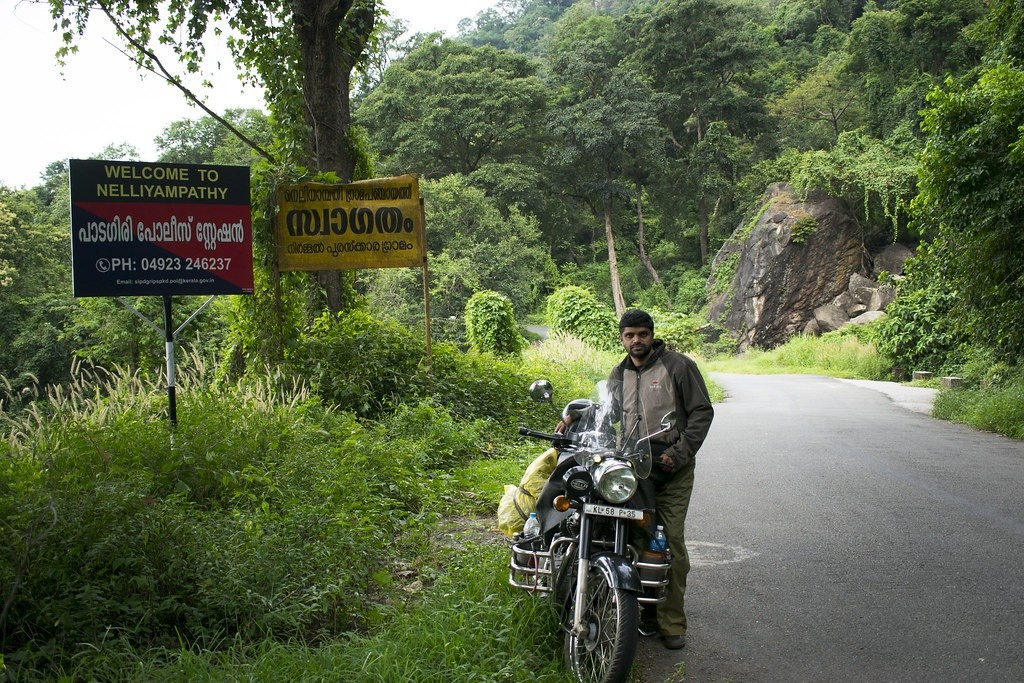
[505,379,676,683]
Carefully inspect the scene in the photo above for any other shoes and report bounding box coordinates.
[662,635,685,649]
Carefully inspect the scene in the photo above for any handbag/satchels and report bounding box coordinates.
[497,448,557,539]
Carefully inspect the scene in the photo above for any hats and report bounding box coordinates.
[619,310,654,331]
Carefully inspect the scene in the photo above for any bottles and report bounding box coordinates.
[650,525,666,552]
[524,513,541,540]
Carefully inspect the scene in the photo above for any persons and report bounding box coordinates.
[555,309,714,648]
